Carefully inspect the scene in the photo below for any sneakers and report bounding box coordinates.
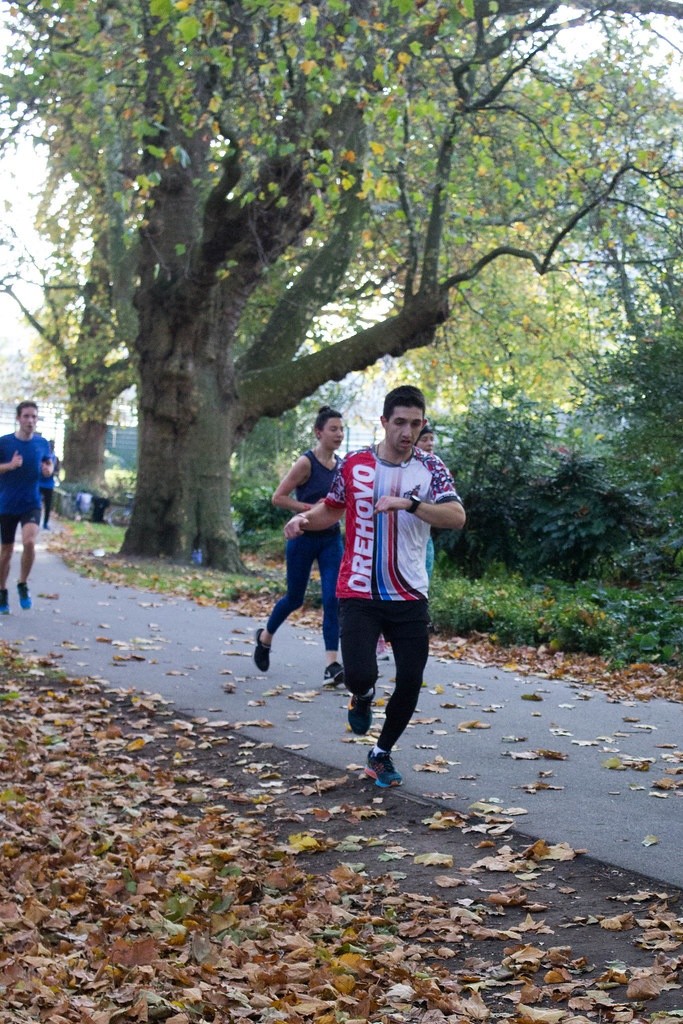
[363,749,403,789]
[17,580,33,610]
[0,588,10,615]
[254,628,273,671]
[348,685,375,735]
[323,660,346,685]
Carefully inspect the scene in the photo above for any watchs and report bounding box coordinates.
[406,496,421,513]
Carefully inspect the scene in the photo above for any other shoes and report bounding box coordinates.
[42,524,49,529]
[376,636,390,660]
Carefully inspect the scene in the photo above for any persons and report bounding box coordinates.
[40,440,59,528]
[283,386,467,787]
[0,400,53,614]
[254,406,344,688]
